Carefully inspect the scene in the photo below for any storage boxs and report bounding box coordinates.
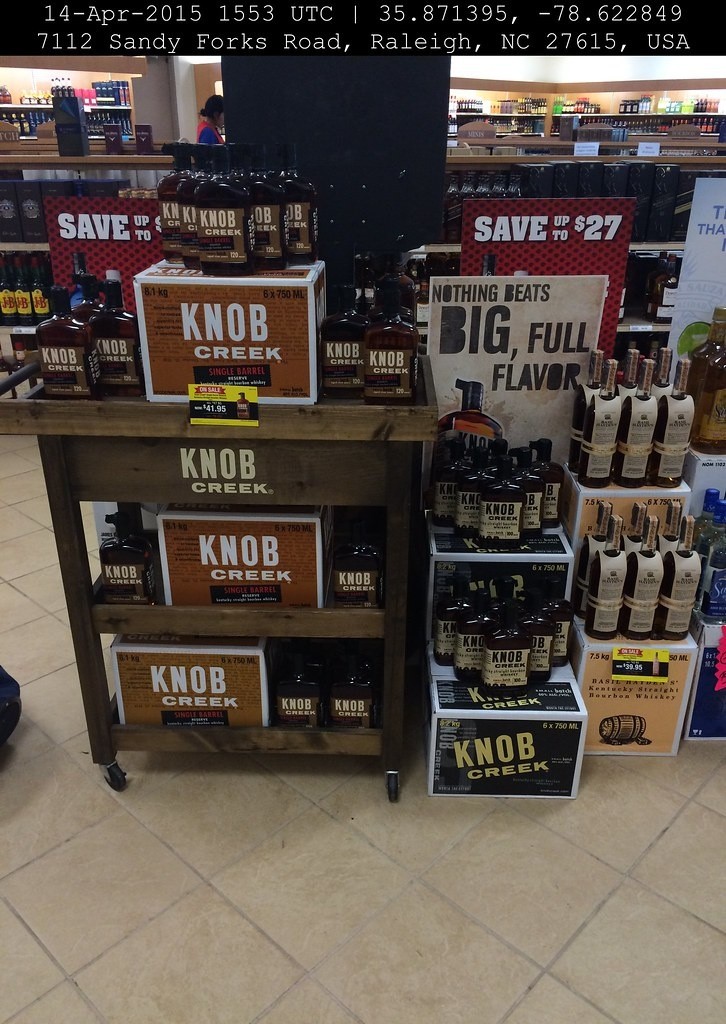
[564,464,693,585]
[425,532,573,638]
[157,506,328,607]
[568,613,701,756]
[683,447,726,519]
[425,663,590,800]
[135,124,154,155]
[53,96,89,157]
[559,116,579,142]
[110,634,271,727]
[105,124,124,154]
[684,613,726,740]
[132,256,326,405]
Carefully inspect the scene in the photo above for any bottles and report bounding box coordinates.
[490,98,546,114]
[649,359,695,487]
[660,501,682,559]
[694,499,726,612]
[576,502,613,619]
[624,502,647,553]
[691,351,726,455]
[658,96,719,113]
[0,250,49,324]
[569,349,604,471]
[552,126,559,132]
[644,252,677,323]
[445,175,520,243]
[692,489,720,550]
[616,360,659,488]
[686,306,726,401]
[481,255,495,277]
[651,348,673,404]
[85,112,134,135]
[617,348,640,408]
[585,515,627,639]
[650,516,701,641]
[553,96,600,114]
[51,77,74,97]
[3,112,52,135]
[448,117,545,133]
[701,537,726,624]
[581,117,725,133]
[618,515,663,640]
[457,99,483,112]
[619,97,653,113]
[69,252,101,311]
[577,359,621,488]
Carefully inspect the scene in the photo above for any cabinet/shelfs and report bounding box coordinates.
[0,354,437,802]
[448,112,547,137]
[549,113,724,138]
[0,104,134,140]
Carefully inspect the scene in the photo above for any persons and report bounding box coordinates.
[196,95,225,144]
